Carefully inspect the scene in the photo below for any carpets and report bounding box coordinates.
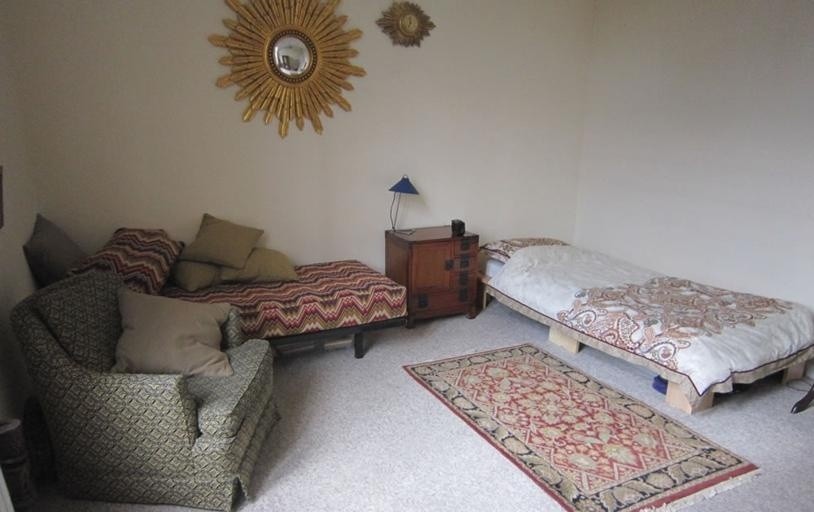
[401,341,762,512]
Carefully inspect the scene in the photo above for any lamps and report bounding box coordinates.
[388,173,420,236]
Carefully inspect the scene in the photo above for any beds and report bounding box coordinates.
[478,237,814,415]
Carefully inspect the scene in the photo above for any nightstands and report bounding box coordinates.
[385,224,480,330]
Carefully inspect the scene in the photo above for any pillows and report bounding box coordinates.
[109,285,235,379]
[213,247,297,283]
[476,235,569,263]
[171,259,218,292]
[22,214,86,280]
[178,212,264,269]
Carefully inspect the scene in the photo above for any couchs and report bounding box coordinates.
[12,269,282,511]
[86,225,404,357]
